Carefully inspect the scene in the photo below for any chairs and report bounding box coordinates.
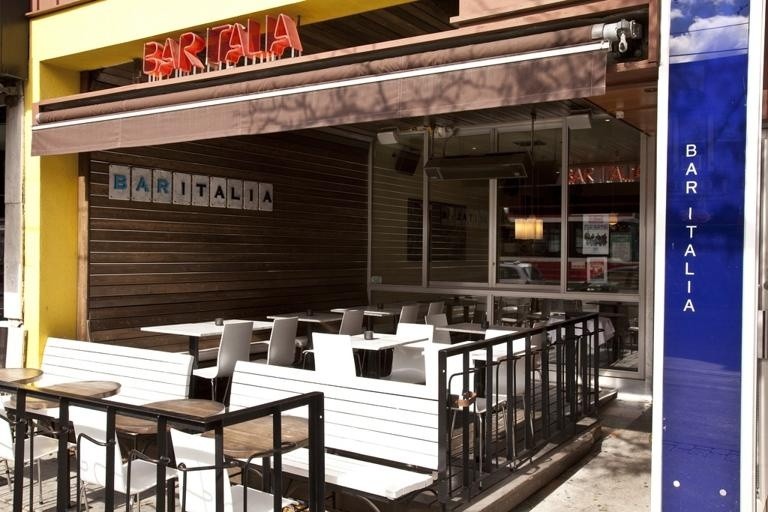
[169,428,300,511]
[192,321,253,401]
[484,328,534,453]
[424,313,452,344]
[381,322,434,383]
[422,343,507,490]
[329,300,546,335]
[69,406,178,511]
[312,332,357,376]
[254,317,298,367]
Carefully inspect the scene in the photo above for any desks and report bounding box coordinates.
[138,318,274,371]
[1,362,121,511]
[546,314,614,395]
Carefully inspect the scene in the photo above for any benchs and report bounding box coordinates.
[227,360,438,500]
[34,337,193,401]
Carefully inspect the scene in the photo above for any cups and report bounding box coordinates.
[307,309,312,316]
[377,303,383,309]
[364,331,373,340]
[215,318,223,326]
[481,321,489,329]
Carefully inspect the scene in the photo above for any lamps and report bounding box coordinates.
[513,138,545,243]
